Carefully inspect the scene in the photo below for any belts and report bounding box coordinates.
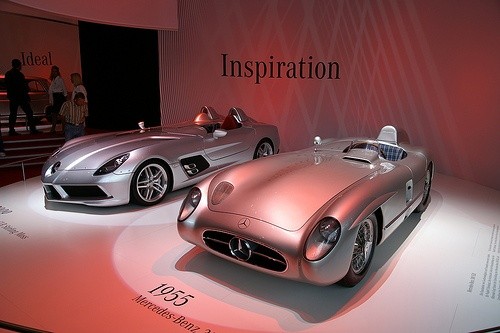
[67,122,85,127]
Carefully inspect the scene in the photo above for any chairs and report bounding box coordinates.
[365,143,404,161]
[194,113,210,121]
[221,115,239,129]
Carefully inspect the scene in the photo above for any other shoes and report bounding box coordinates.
[50,129,56,133]
[30,130,43,134]
[9,131,21,135]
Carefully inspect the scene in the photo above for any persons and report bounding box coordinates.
[5,59,42,136]
[70,72,88,103]
[49,66,67,134]
[59,92,88,142]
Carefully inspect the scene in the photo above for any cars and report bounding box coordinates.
[0,75,73,123]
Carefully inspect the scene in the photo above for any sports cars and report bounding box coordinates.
[176,124,434,289]
[40,106,281,210]
